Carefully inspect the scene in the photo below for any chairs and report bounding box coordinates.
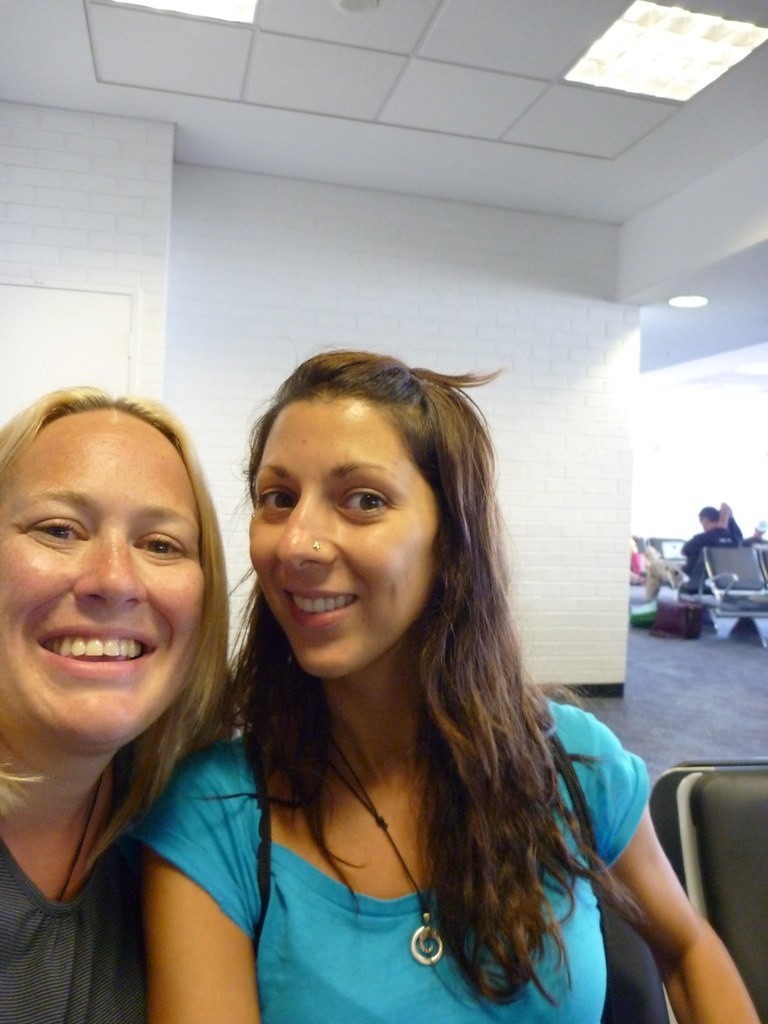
[681,547,768,648]
[602,759,768,1024]
[634,537,686,566]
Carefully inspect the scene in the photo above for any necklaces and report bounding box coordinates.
[327,735,443,966]
[57,773,103,901]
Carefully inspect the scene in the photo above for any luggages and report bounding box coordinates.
[650,599,703,639]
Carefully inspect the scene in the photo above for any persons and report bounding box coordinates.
[645,503,743,603]
[0,386,236,1024]
[125,349,762,1024]
[742,521,768,547]
